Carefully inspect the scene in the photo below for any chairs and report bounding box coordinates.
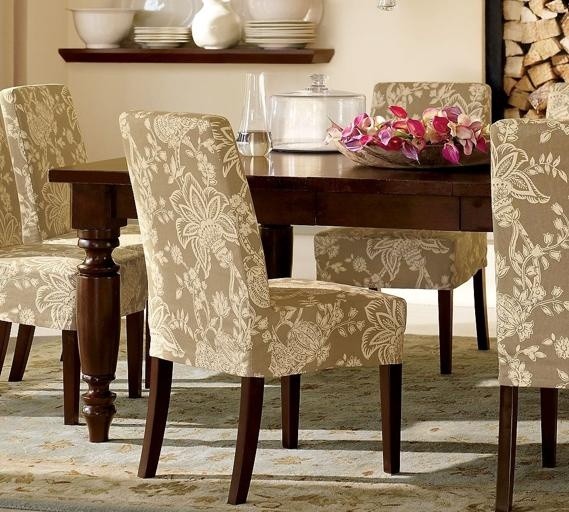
[309,81,495,377]
[115,104,411,507]
[483,115,569,511]
[543,81,569,124]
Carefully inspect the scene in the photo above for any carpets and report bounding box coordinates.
[0,330,567,512]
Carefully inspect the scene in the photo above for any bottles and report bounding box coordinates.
[235,70,273,156]
[192,0,244,50]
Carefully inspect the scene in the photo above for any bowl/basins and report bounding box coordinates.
[65,8,139,51]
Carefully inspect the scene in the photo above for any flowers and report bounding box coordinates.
[321,102,497,168]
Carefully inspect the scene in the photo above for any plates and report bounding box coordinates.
[242,17,318,50]
[132,22,191,49]
